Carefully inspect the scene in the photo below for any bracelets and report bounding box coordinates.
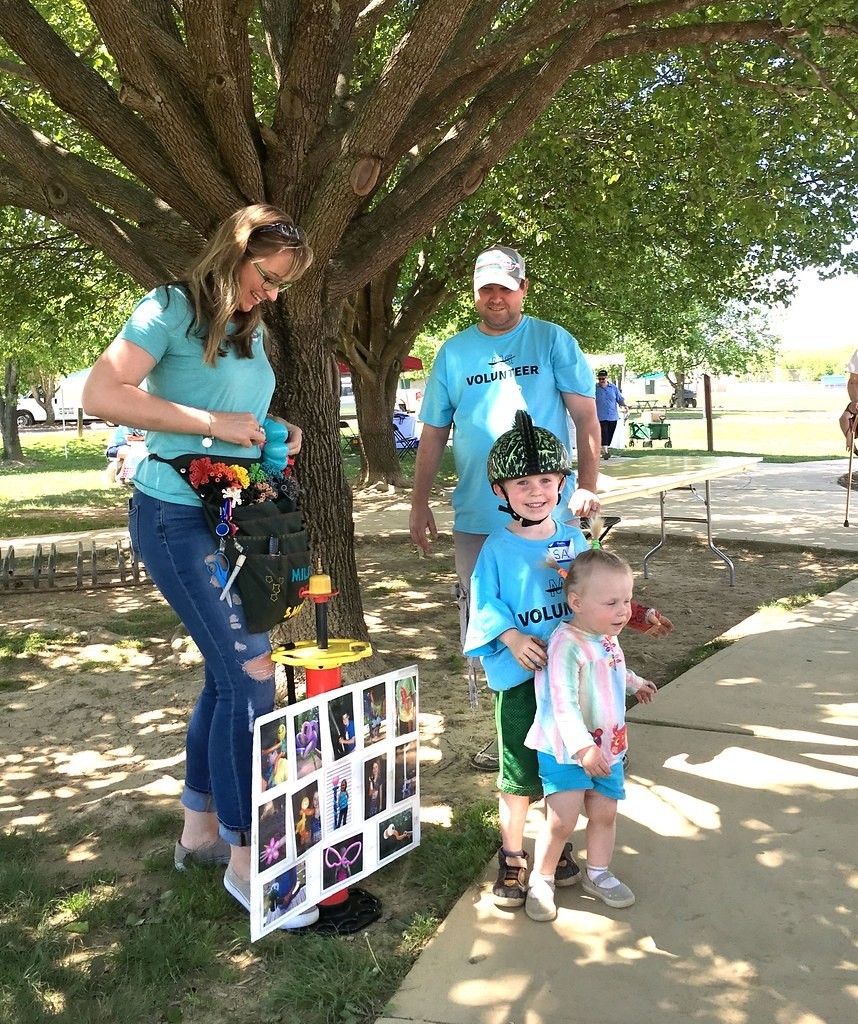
[202,411,214,448]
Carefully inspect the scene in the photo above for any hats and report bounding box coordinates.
[473,246,525,293]
[597,370,607,376]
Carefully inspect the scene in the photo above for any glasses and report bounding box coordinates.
[249,254,292,293]
[259,222,305,243]
[598,378,605,380]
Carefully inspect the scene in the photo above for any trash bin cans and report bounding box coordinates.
[645,384,651,394]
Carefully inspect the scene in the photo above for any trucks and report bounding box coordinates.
[14,378,123,429]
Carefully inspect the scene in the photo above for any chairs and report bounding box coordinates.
[580,517,621,543]
[393,424,418,462]
[339,422,363,454]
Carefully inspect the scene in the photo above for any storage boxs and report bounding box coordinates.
[629,423,670,439]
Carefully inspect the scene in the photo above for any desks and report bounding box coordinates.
[339,409,416,420]
[636,400,658,412]
[575,455,763,587]
[394,416,416,448]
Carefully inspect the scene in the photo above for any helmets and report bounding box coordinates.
[487,409,572,487]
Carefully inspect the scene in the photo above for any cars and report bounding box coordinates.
[338,354,425,422]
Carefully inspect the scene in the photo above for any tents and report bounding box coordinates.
[335,356,427,411]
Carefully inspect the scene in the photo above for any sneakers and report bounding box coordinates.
[223,861,320,928]
[493,847,530,908]
[174,837,233,873]
[554,842,583,887]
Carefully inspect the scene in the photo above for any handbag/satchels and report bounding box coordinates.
[171,451,311,634]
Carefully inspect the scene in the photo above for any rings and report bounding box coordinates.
[591,509,597,512]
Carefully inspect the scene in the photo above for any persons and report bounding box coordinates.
[408,247,603,773]
[463,408,674,910]
[525,549,658,921]
[847,348,858,402]
[596,370,627,460]
[105,425,147,483]
[261,712,355,791]
[82,204,322,934]
[394,677,416,732]
[368,757,383,817]
[312,792,322,842]
[337,779,350,828]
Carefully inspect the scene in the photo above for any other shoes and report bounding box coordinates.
[602,453,611,460]
[581,866,635,908]
[525,875,557,921]
